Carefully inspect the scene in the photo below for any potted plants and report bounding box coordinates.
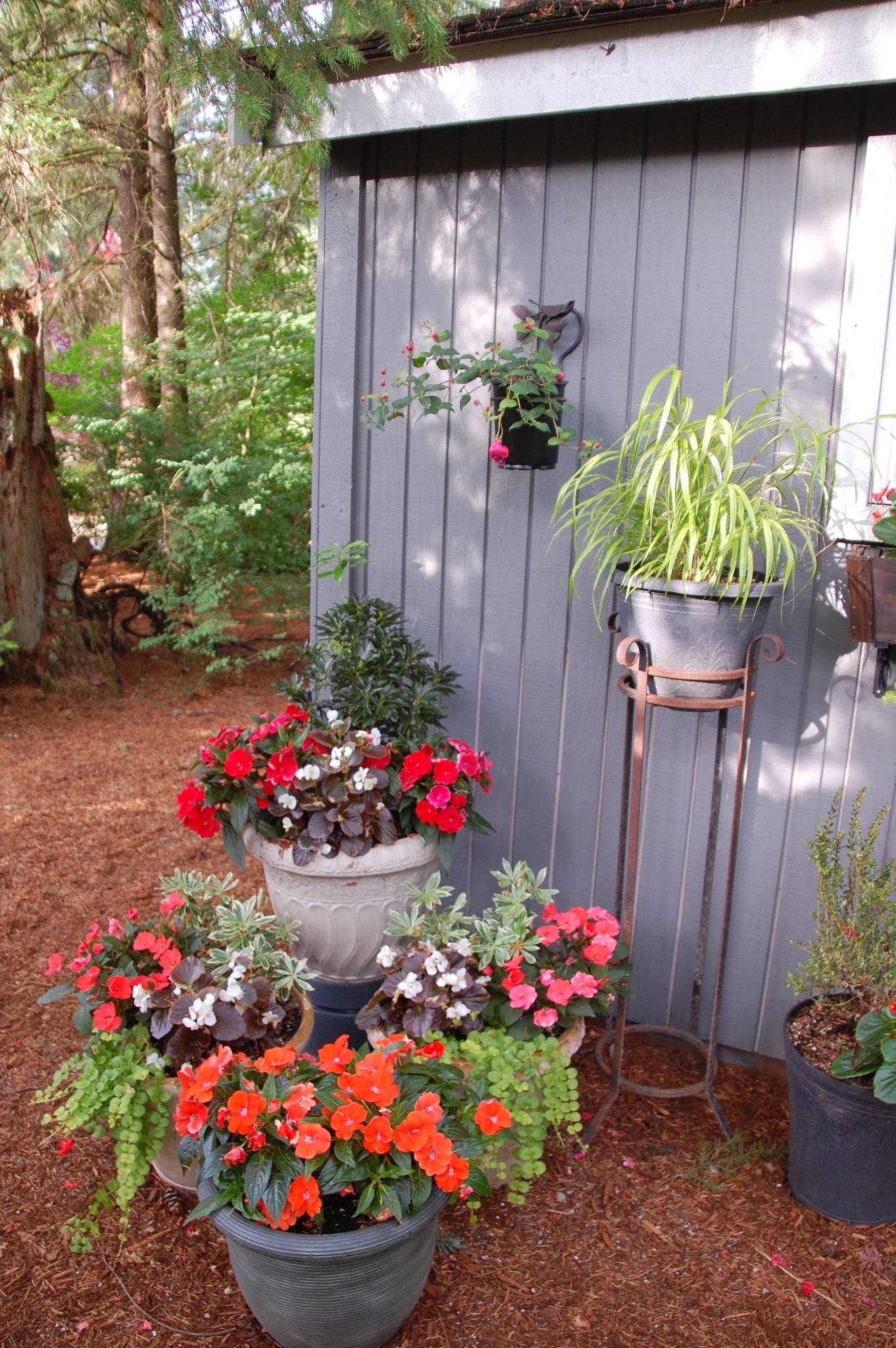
[548,365,896,713]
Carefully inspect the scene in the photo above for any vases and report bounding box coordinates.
[198,1171,452,1348]
[142,989,311,1195]
[453,1016,591,1185]
[243,824,440,982]
[490,380,568,469]
[846,541,896,645]
[783,992,896,1222]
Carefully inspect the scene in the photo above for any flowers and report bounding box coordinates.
[870,480,895,559]
[786,784,895,1105]
[29,594,633,1258]
[361,308,601,466]
